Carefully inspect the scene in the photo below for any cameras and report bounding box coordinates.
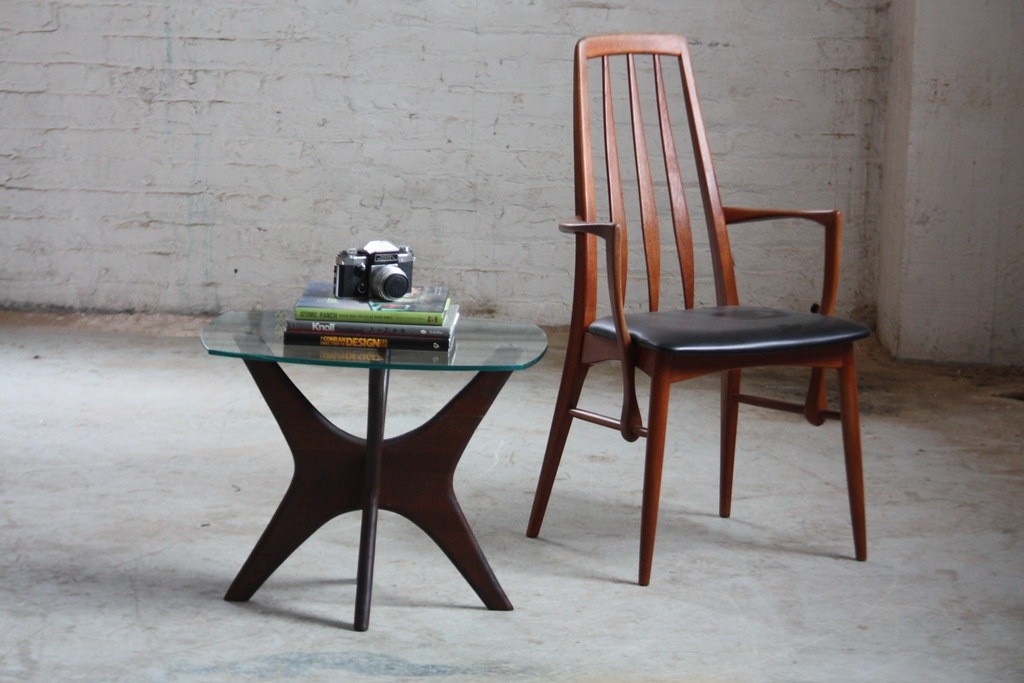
[333,240,415,302]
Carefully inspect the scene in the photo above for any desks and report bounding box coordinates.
[198,308,548,630]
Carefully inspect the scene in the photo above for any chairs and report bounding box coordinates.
[525,32,867,587]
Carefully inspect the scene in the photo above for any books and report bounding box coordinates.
[284,278,459,351]
[282,342,457,366]
